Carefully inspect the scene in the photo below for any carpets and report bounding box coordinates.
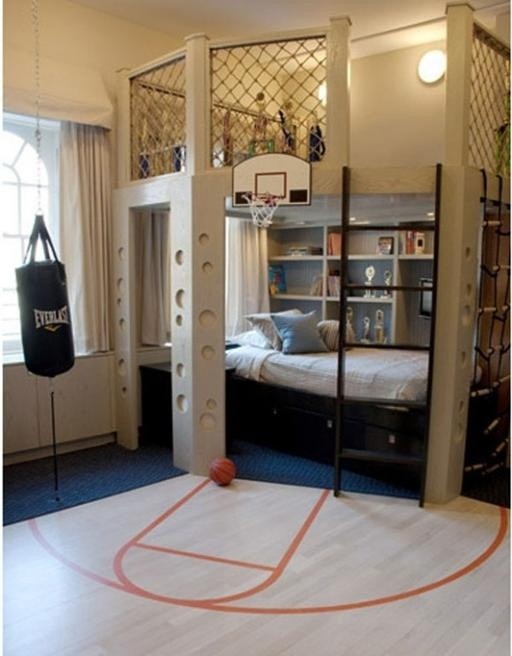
[461,468,510,509]
[1,435,189,526]
[224,437,422,500]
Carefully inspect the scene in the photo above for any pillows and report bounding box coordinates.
[231,306,355,355]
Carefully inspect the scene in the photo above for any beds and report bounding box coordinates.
[225,336,483,486]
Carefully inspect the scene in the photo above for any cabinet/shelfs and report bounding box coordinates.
[266,221,434,346]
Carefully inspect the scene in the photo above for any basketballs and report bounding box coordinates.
[209,458,237,486]
